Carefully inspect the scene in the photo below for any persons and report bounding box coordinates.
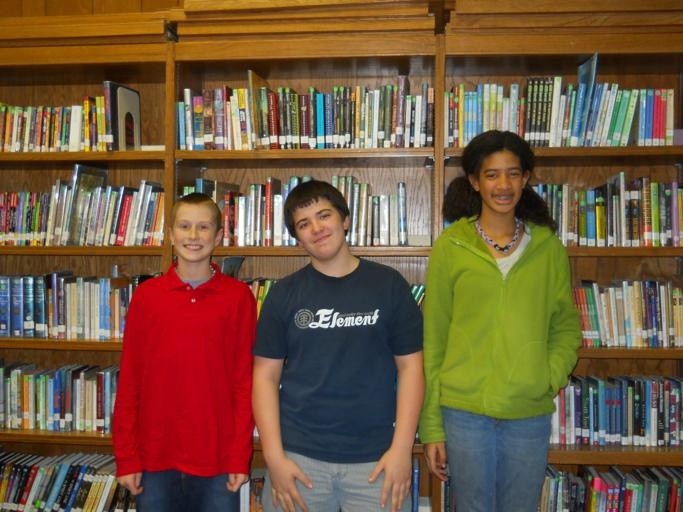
[416,129,584,511]
[250,180,426,512]
[107,191,256,511]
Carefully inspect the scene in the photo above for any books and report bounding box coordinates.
[444,463,456,511]
[444,52,683,147]
[221,256,431,512]
[176,68,432,247]
[1,164,162,512]
[532,172,683,512]
[0,81,164,152]
